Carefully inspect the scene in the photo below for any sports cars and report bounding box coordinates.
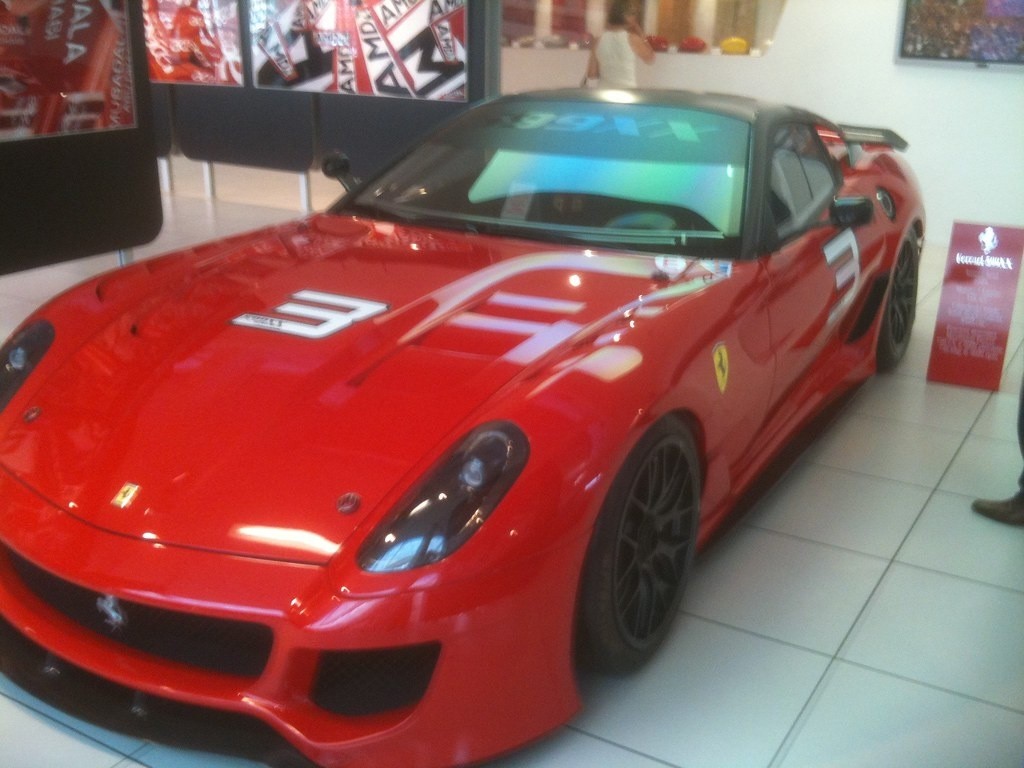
[3,85,925,768]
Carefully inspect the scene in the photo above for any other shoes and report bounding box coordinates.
[972,489,1024,526]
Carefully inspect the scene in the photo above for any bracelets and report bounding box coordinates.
[643,39,648,43]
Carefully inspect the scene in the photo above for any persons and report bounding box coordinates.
[972,372,1024,527]
[586,0,657,89]
